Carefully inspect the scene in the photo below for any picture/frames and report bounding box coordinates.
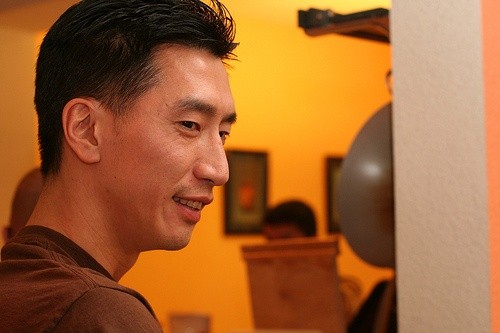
[221,151,269,233]
[326,157,342,240]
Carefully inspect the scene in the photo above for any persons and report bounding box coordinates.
[0,167,40,243]
[262,200,317,243]
[342,273,399,333]
[0,0,241,333]
